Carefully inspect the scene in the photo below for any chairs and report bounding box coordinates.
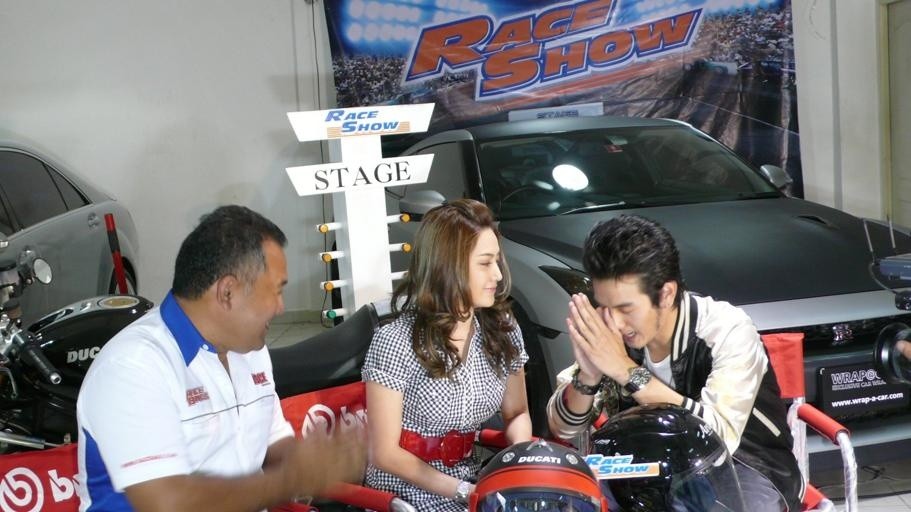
[277,380,573,512]
[760,332,860,512]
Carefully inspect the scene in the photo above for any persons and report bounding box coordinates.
[73,205,369,512]
[544,214,803,512]
[357,197,536,512]
[894,340,911,359]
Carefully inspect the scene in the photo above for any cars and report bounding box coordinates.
[2,138,141,329]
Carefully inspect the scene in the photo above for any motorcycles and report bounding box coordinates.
[2,245,394,512]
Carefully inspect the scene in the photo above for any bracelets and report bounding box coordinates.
[572,366,607,396]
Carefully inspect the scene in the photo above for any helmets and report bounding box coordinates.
[593,403,745,511]
[469,441,607,512]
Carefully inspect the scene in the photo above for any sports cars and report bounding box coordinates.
[331,115,909,502]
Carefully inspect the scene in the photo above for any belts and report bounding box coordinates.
[399,429,476,468]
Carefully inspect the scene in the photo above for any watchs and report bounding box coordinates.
[622,364,652,398]
[451,478,474,504]
